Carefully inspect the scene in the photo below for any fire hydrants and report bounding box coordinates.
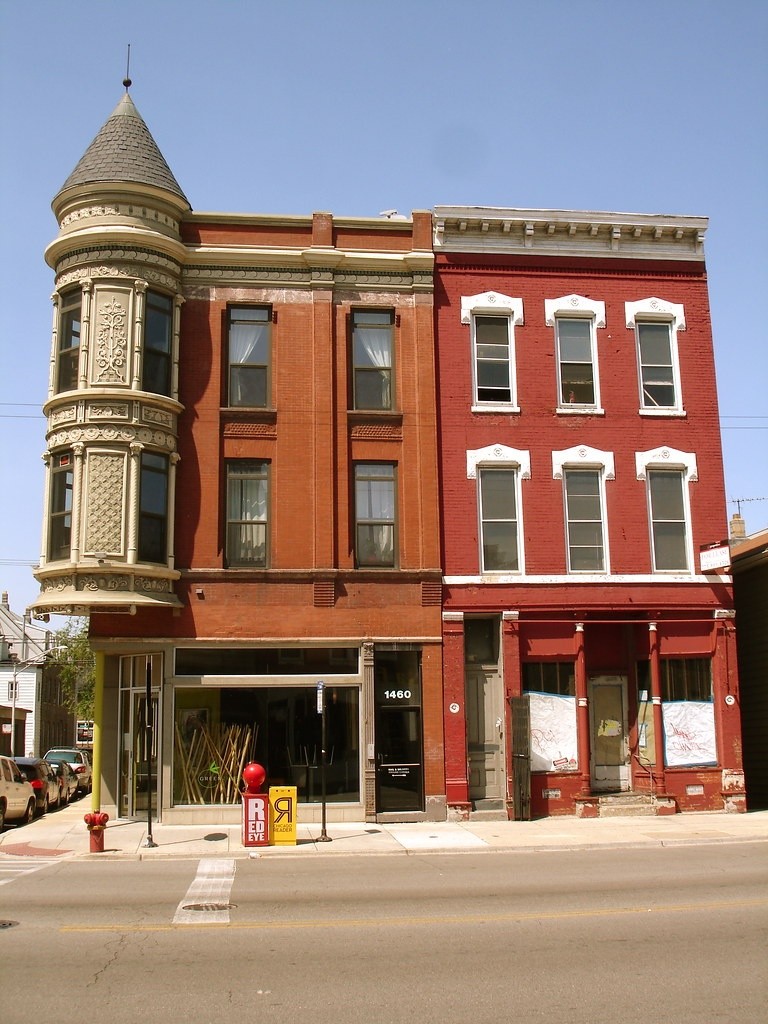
[83,809,109,854]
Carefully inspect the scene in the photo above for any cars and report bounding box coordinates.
[43,757,81,807]
[10,756,63,815]
[0,755,37,834]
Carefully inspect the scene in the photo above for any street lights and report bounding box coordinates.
[11,646,68,756]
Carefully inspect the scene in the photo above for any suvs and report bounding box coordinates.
[41,749,91,792]
